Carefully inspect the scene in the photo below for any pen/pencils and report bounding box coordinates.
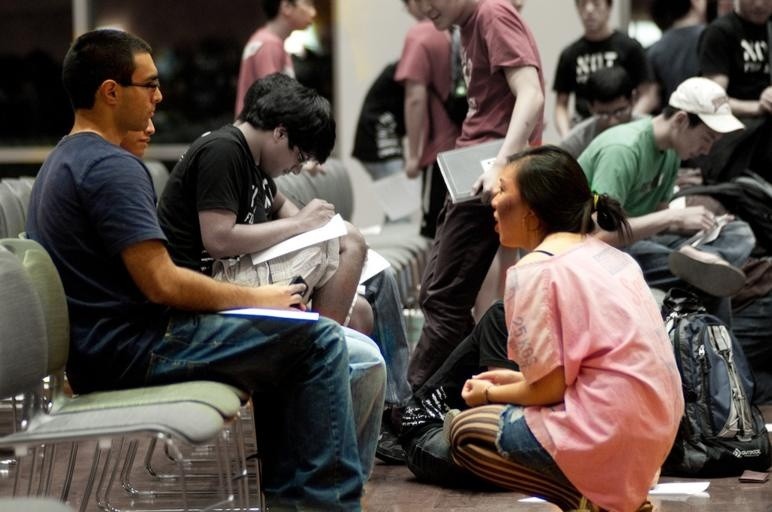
[292,195,306,207]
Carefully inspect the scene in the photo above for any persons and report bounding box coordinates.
[157,82,377,340]
[234,73,415,467]
[119,116,387,482]
[352,58,406,179]
[232,0,318,124]
[25,28,365,512]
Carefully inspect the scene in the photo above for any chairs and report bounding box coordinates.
[141,159,173,208]
[0,175,36,241]
[0,238,251,509]
[274,157,429,311]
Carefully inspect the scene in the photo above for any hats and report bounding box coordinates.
[667,75,749,137]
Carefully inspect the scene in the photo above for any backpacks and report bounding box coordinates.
[657,285,772,471]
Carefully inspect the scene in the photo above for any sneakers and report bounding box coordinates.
[376,425,411,466]
[665,243,748,301]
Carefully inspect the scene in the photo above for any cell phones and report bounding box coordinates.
[288,275,308,309]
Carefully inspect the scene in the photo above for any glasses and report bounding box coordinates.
[119,75,164,92]
[589,105,632,120]
[292,141,311,169]
